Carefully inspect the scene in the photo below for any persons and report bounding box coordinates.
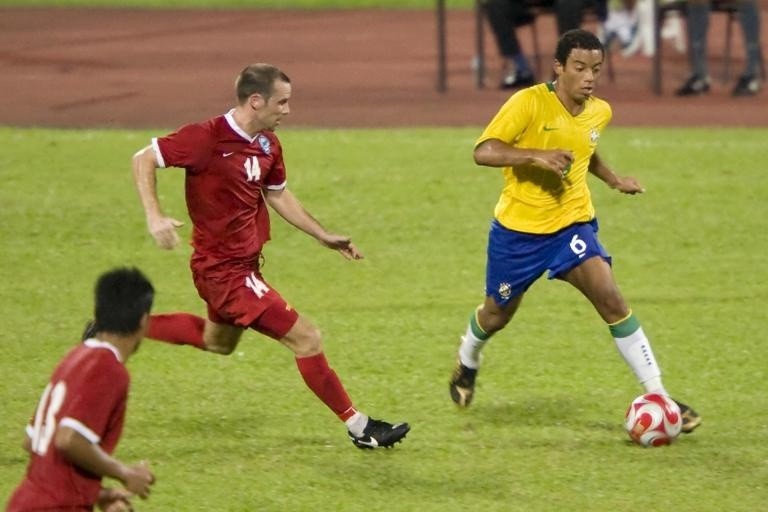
[80,61,411,450]
[476,1,610,90]
[450,28,711,427]
[673,0,764,98]
[2,263,159,512]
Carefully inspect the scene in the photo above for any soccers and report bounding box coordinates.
[626,395,681,447]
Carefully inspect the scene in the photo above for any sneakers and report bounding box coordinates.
[665,395,702,435]
[498,68,536,90]
[346,416,411,451]
[732,72,764,97]
[450,356,480,409]
[676,73,712,97]
